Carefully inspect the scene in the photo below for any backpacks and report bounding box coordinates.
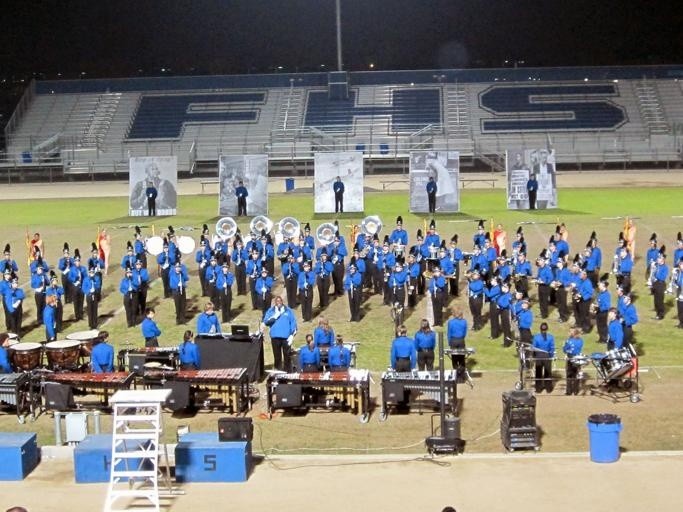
[7,333,20,342]
[8,342,43,371]
[600,347,634,379]
[2,339,19,347]
[177,234,196,255]
[65,329,100,358]
[144,236,165,256]
[43,339,82,369]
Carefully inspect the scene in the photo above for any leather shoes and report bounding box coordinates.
[287,335,294,346]
[273,311,282,319]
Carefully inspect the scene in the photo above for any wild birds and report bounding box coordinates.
[232,325,248,335]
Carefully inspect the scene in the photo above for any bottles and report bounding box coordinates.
[516,224,682,305]
[320,216,513,287]
[123,223,311,273]
[4,242,98,284]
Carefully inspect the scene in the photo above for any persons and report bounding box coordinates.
[313,316,334,395]
[526,173,540,212]
[141,306,163,347]
[333,175,346,213]
[235,181,249,217]
[129,161,177,211]
[391,324,417,395]
[404,248,420,307]
[42,293,59,343]
[221,170,251,201]
[593,272,611,343]
[345,217,408,322]
[176,329,200,375]
[670,232,681,329]
[300,261,316,322]
[91,330,113,373]
[0,331,19,374]
[0,221,299,330]
[465,219,533,348]
[427,261,448,327]
[414,218,464,308]
[146,182,158,217]
[414,319,436,371]
[606,307,624,390]
[650,244,669,319]
[614,276,638,357]
[314,250,334,307]
[563,328,583,396]
[423,152,456,212]
[610,231,633,304]
[425,176,439,215]
[316,221,348,296]
[531,322,555,393]
[507,152,530,210]
[447,307,468,383]
[623,219,637,263]
[262,295,298,373]
[197,302,223,337]
[531,222,602,333]
[300,334,321,410]
[300,222,315,304]
[328,334,351,407]
[532,150,558,209]
[512,298,535,370]
[645,233,660,295]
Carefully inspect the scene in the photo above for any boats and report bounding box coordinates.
[46,385,74,411]
[163,381,191,410]
[276,384,302,408]
[382,382,404,402]
[218,417,253,440]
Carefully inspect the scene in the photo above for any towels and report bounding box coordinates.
[22,152,32,163]
[356,143,365,154]
[586,413,621,463]
[285,178,294,192]
[380,143,389,154]
